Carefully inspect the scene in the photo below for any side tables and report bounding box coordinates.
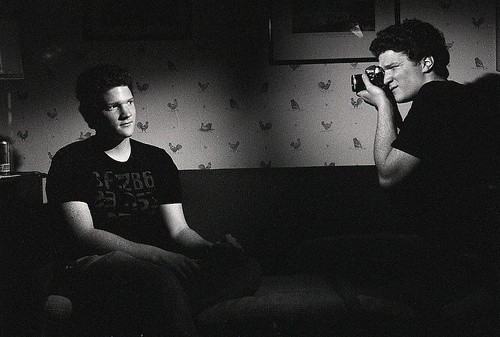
[0,169,43,296]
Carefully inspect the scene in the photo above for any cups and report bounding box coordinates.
[0,141,10,175]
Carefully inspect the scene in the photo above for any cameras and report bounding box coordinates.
[351,65,385,92]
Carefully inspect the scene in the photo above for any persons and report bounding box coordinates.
[45,63,244,336]
[326,17,499,190]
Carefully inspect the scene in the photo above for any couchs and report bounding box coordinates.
[34,166,452,337]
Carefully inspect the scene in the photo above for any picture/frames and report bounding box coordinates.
[82,0,199,47]
[268,1,401,66]
